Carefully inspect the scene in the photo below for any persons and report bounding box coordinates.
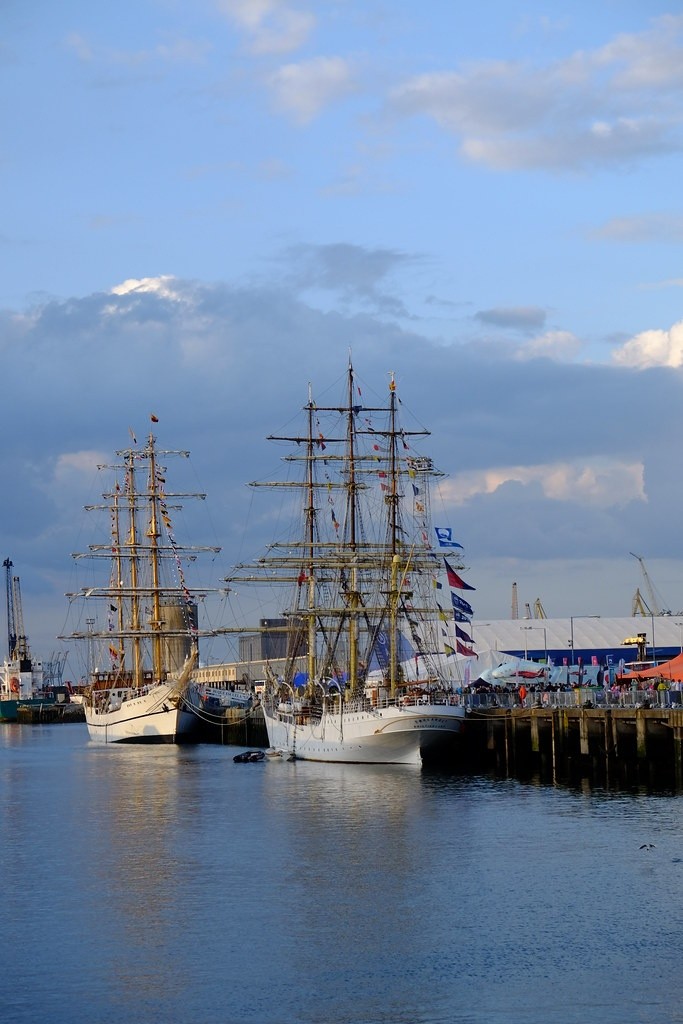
[387,679,683,708]
[126,679,165,699]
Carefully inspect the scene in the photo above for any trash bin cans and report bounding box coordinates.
[573,686,602,705]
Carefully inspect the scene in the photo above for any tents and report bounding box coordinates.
[365,650,683,689]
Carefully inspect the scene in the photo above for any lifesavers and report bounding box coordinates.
[519,686,526,699]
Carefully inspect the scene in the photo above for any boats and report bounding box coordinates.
[0,554,71,722]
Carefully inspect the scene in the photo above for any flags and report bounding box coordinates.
[102,414,197,660]
[297,384,478,658]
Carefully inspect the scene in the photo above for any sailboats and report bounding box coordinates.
[220,345,480,765]
[56,414,232,745]
